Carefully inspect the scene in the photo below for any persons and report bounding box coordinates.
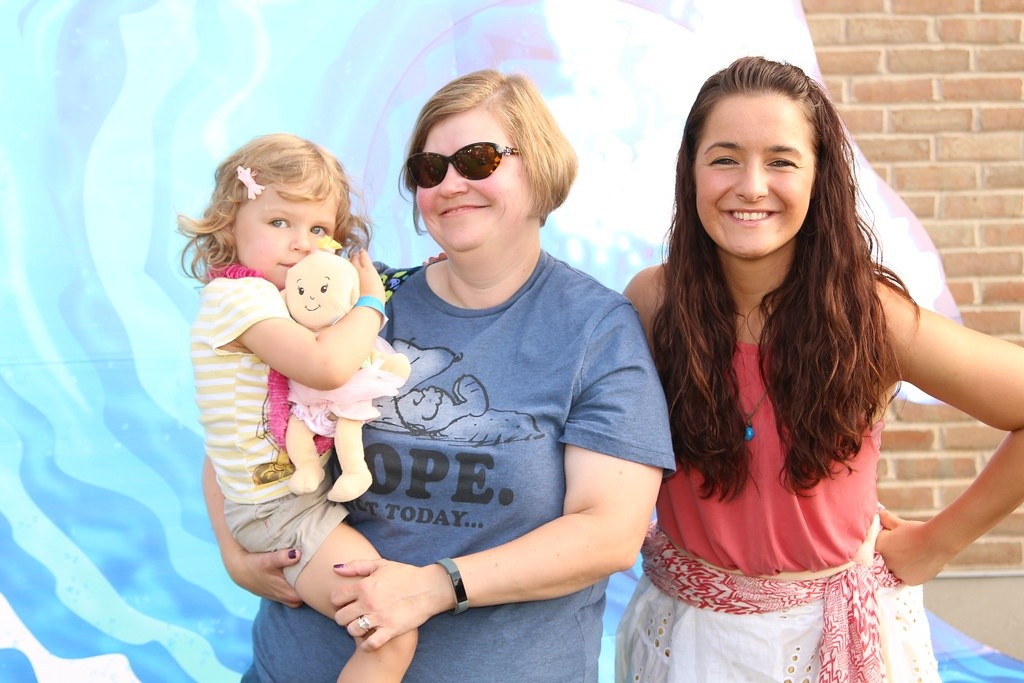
[203,71,678,683]
[623,56,1024,683]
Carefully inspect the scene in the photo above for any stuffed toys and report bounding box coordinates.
[203,235,411,503]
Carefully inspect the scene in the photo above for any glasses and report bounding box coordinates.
[406,142,519,188]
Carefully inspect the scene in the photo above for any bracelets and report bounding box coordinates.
[434,557,469,617]
[353,293,389,331]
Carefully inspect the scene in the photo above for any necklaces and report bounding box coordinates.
[737,380,769,441]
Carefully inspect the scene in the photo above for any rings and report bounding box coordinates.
[357,617,372,634]
